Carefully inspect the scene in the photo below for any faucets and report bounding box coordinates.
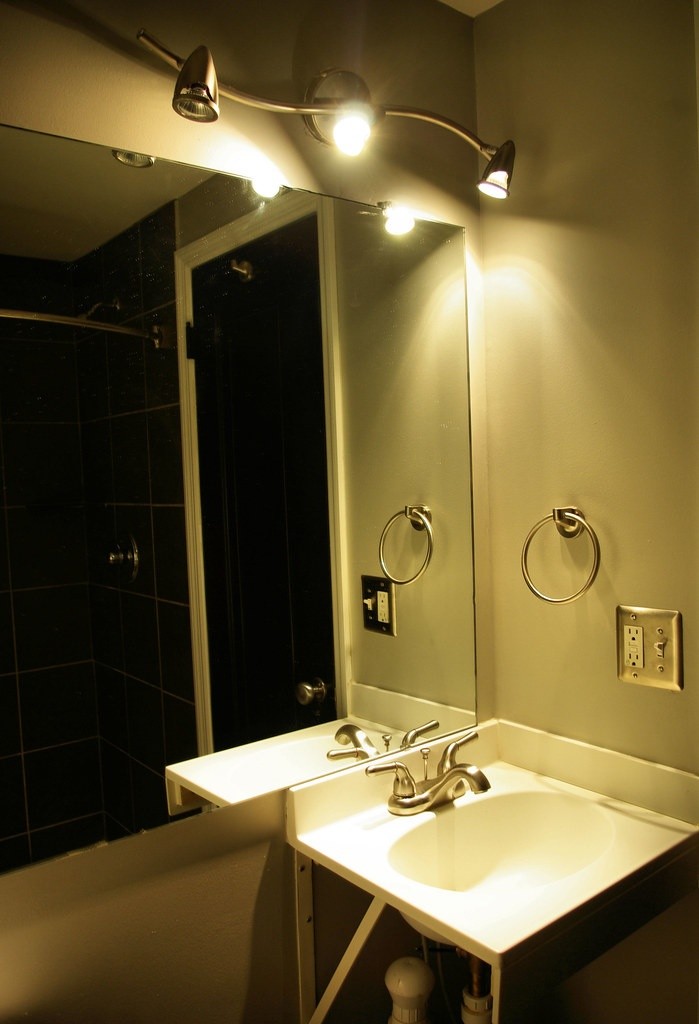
[364,732,491,817]
[326,720,441,763]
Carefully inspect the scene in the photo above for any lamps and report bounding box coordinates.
[136,28,516,199]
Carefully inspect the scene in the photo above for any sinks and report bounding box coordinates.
[228,734,362,785]
[386,790,613,895]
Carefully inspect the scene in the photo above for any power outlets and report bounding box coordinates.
[359,575,397,637]
[615,606,684,691]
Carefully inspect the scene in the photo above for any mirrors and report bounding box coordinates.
[1,127,482,876]
